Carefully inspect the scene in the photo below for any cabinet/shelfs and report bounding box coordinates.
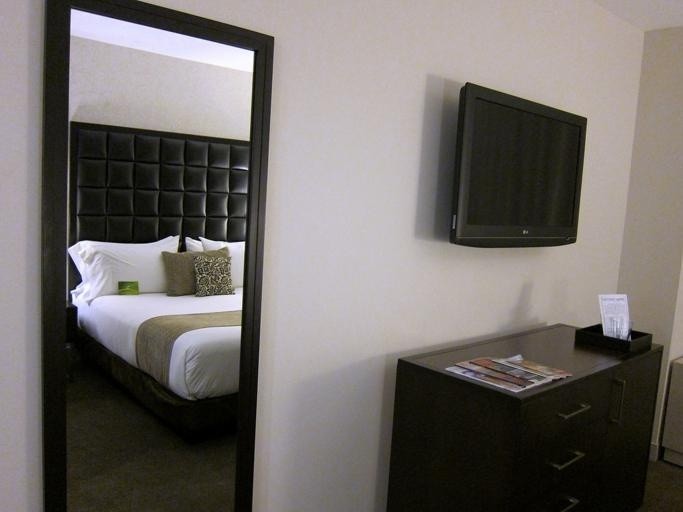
[385,322,665,510]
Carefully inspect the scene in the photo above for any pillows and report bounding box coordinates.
[67,234,246,311]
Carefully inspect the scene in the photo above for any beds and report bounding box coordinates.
[67,284,251,454]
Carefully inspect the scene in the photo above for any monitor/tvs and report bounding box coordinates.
[449,82,588,248]
